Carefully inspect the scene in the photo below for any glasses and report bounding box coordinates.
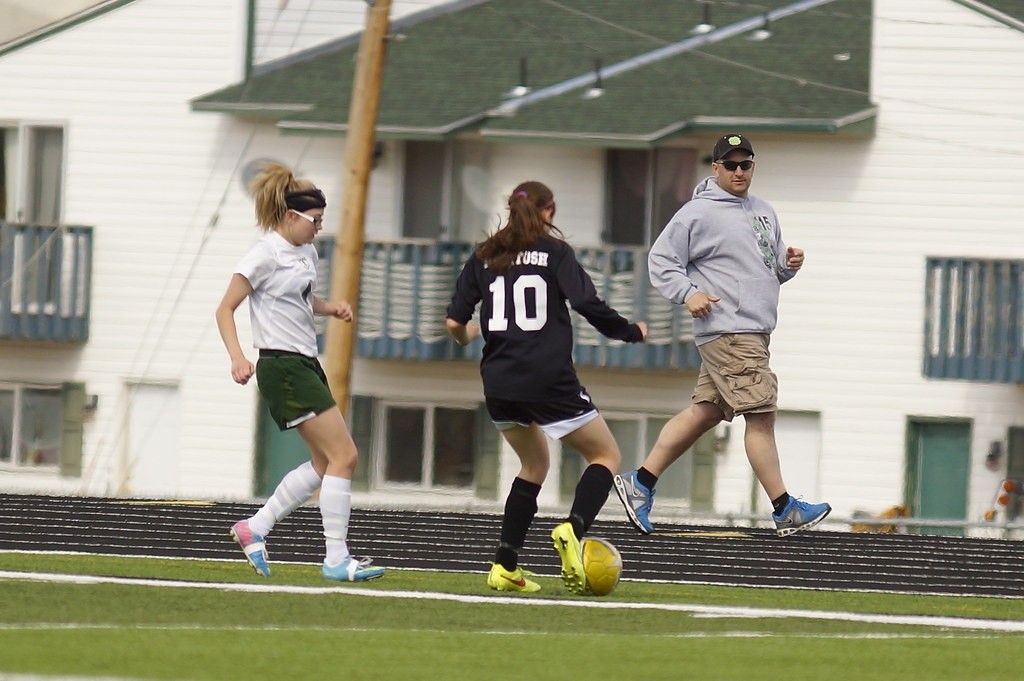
[288,208,323,227]
[715,160,754,171]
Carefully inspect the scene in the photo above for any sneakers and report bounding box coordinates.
[613,469,657,538]
[772,495,832,539]
[486,563,542,593]
[321,553,386,583]
[230,518,272,579]
[550,521,586,596]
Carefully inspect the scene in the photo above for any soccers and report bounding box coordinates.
[574,535,624,596]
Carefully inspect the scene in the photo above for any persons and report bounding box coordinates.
[613,133,832,538]
[216,162,386,582]
[447,181,650,592]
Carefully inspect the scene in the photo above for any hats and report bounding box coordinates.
[712,133,755,163]
